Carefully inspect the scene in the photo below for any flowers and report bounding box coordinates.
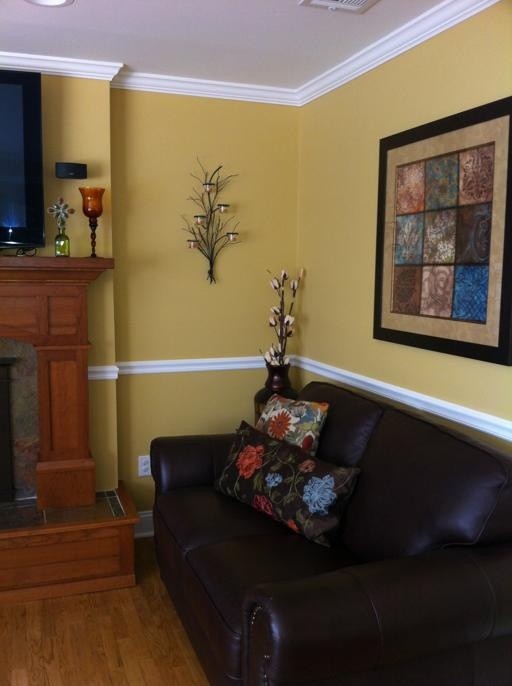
[47,196,76,225]
[258,264,312,366]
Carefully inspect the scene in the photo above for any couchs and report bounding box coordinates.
[149,378,512,684]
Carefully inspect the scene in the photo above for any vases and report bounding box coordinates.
[254,363,301,399]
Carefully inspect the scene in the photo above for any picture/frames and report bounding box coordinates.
[371,94,512,370]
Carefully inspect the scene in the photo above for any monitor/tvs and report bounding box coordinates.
[0,69,46,248]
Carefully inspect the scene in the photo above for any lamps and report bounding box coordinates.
[181,156,243,282]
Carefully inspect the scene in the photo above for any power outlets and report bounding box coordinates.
[137,453,151,478]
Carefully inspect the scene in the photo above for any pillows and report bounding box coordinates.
[210,418,365,549]
[254,388,330,456]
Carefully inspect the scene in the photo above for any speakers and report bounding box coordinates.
[55,162,88,179]
